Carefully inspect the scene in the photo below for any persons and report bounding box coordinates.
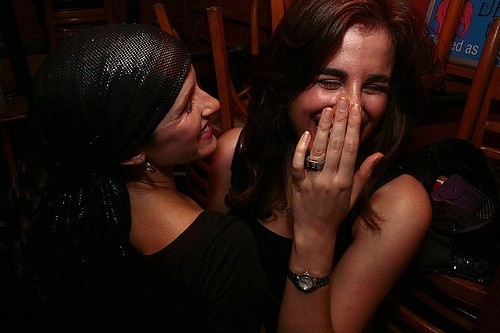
[205,0,437,333]
[0,20,264,333]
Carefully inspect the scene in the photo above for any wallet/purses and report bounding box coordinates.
[439,174,488,213]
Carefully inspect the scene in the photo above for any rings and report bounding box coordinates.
[305,157,326,173]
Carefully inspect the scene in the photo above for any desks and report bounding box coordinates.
[0,41,210,187]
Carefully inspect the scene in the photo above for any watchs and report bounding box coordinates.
[285,266,332,292]
